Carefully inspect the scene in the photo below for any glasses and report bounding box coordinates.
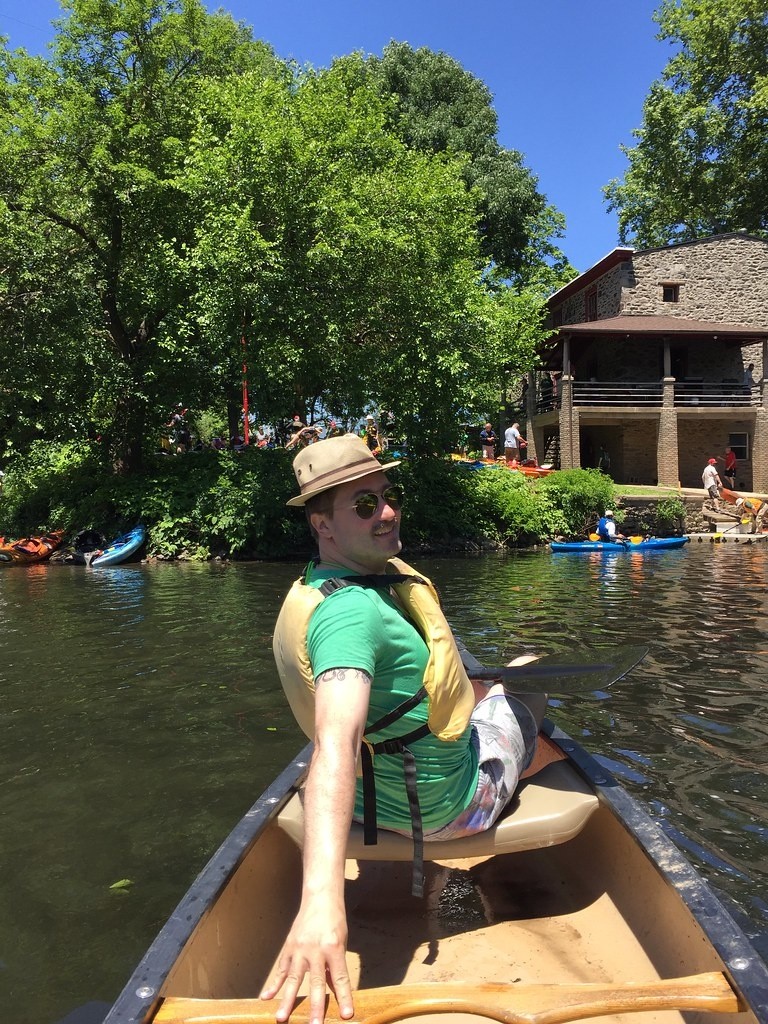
[319,487,403,520]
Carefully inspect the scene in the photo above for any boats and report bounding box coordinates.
[546,537,690,551]
[0,526,64,564]
[50,525,145,568]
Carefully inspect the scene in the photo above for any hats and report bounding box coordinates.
[286,433,402,507]
[259,426,263,429]
[520,379,527,382]
[315,427,323,433]
[707,458,717,464]
[366,415,374,420]
[605,510,613,516]
[294,416,299,419]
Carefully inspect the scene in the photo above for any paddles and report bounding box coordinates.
[463,644,651,696]
[588,532,644,545]
[713,516,752,538]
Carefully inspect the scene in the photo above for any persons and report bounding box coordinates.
[504,423,529,463]
[163,401,394,457]
[259,433,550,1024]
[718,447,737,491]
[596,510,624,543]
[701,459,723,510]
[734,495,768,534]
[598,444,610,475]
[479,423,499,460]
